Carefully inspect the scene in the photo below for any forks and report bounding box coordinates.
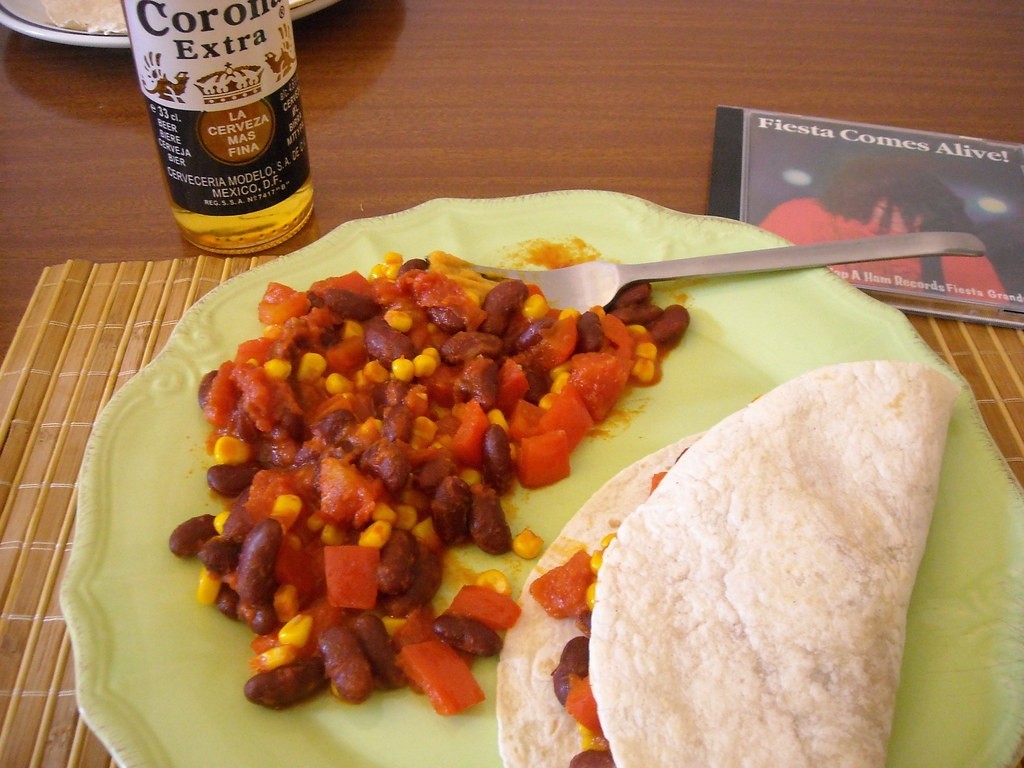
[425,230,987,314]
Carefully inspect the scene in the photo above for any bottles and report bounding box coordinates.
[119,0,314,255]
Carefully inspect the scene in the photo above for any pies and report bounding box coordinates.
[493,360,954,768]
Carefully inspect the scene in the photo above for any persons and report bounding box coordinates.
[754,157,1010,305]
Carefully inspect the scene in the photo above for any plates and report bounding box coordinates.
[0,0,336,48]
[58,188,1024,767]
